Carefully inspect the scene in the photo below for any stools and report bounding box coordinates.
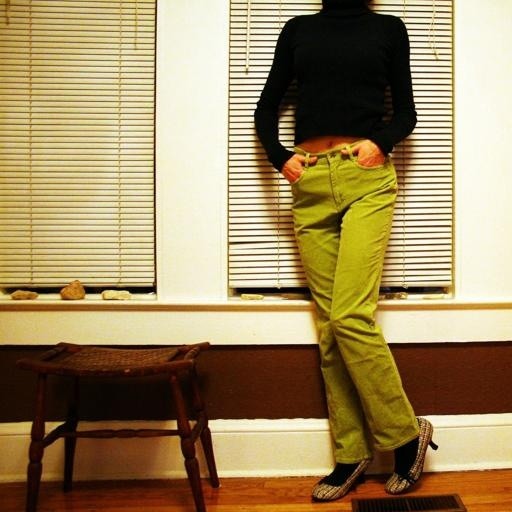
[16,342,221,512]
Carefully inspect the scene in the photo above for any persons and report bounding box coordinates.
[253,3,439,502]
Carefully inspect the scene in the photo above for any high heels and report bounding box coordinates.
[384,417,439,494]
[312,457,372,503]
